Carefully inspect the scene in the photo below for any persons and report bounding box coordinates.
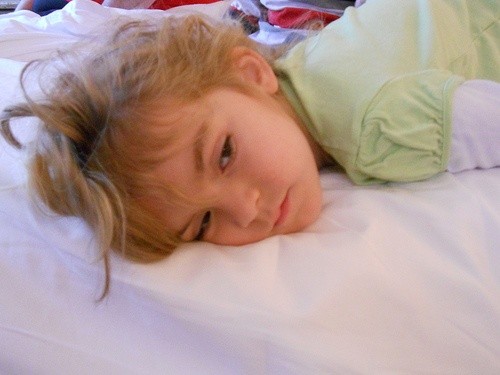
[1,0,500,305]
